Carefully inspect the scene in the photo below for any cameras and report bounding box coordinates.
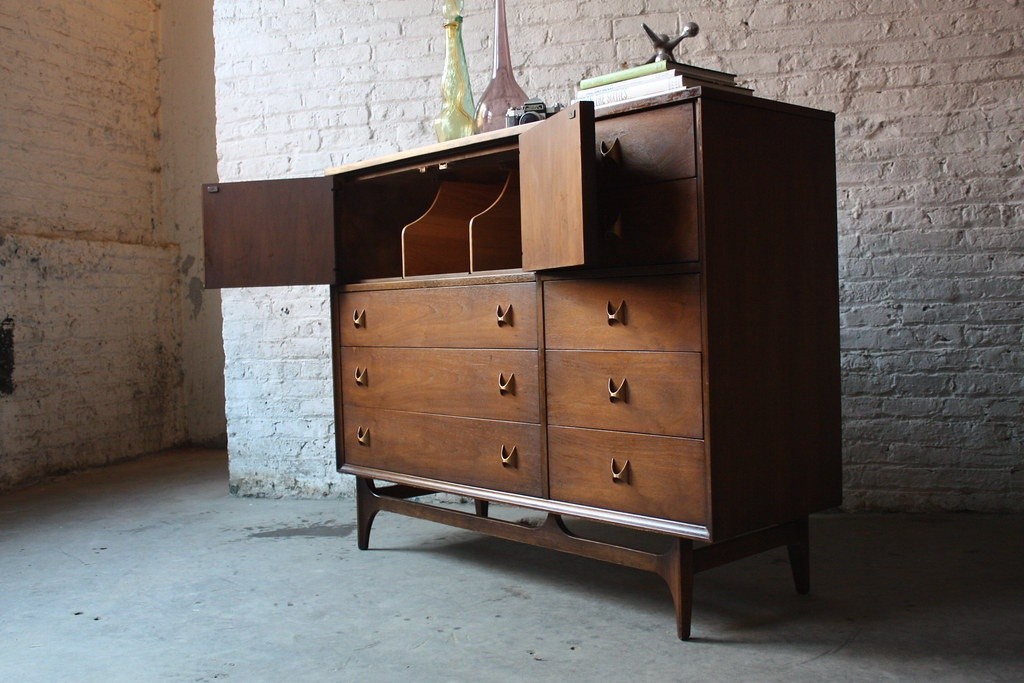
[506,102,564,128]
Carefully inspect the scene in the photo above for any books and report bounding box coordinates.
[570,58,756,112]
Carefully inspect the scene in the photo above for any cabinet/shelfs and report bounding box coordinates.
[202,86,844,641]
